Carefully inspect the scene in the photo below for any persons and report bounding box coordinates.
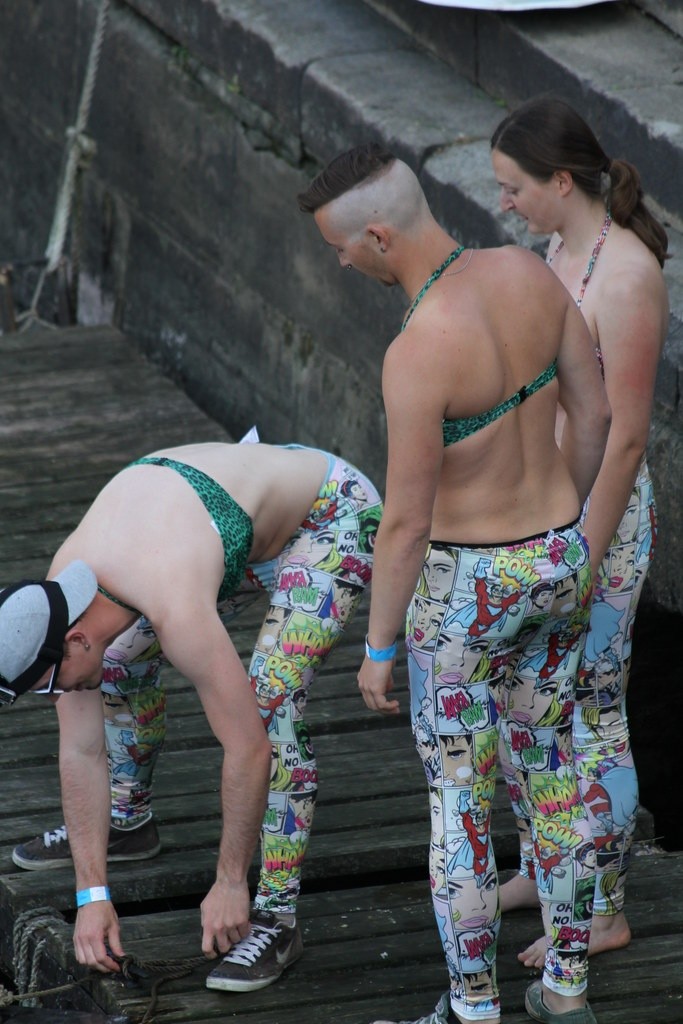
[292,94,668,1024]
[0,443,383,993]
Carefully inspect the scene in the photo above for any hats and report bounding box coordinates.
[0,559,102,708]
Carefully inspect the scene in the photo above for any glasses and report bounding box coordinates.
[28,650,64,702]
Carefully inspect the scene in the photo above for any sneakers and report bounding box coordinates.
[525,980,597,1024]
[207,908,305,992]
[374,990,466,1024]
[12,812,159,871]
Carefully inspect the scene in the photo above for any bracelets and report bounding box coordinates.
[365,634,398,663]
[76,886,111,907]
